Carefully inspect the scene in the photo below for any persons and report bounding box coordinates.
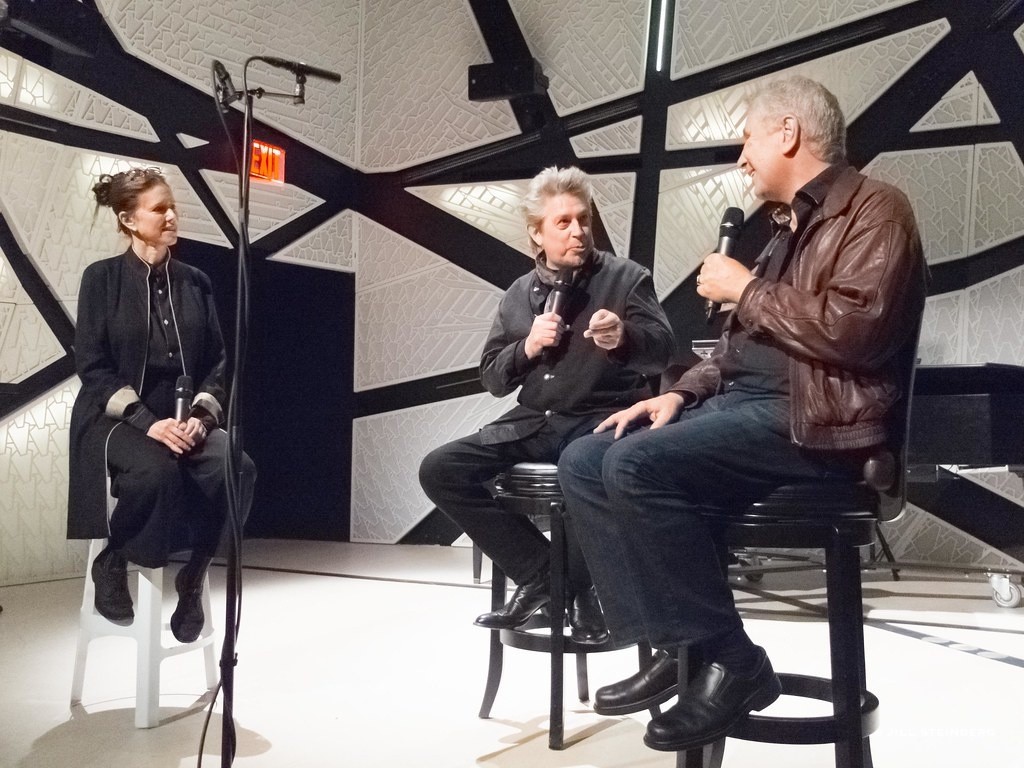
[560,74,931,750]
[418,165,677,645]
[66,167,257,642]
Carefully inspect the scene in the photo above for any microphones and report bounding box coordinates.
[216,63,236,96]
[259,56,341,83]
[172,375,194,459]
[703,207,744,326]
[540,265,575,363]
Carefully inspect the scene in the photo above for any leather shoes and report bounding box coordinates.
[593,650,678,716]
[473,571,551,630]
[642,645,782,751]
[566,588,610,644]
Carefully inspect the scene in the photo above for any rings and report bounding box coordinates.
[696,275,700,285]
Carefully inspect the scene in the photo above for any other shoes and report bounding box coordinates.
[170,565,205,643]
[91,545,135,627]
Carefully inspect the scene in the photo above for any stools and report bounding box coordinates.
[65,539,222,730]
[672,361,919,768]
[479,460,662,750]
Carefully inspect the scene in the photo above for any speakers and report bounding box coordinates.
[467,58,544,103]
